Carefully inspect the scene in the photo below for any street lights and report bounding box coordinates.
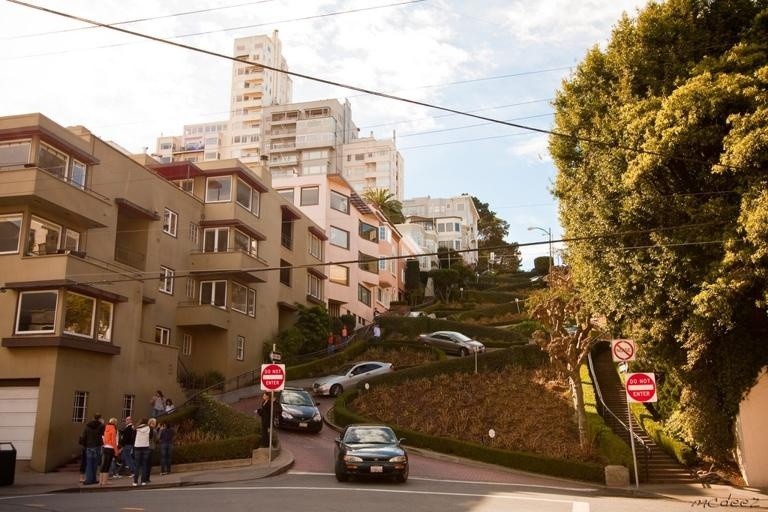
[527,227,552,288]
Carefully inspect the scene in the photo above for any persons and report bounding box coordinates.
[271,393,283,420]
[253,391,273,447]
[164,398,175,414]
[373,323,381,340]
[149,390,165,417]
[74,409,176,487]
[341,324,350,343]
[326,331,337,356]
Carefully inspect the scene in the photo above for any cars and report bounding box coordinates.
[260,386,323,433]
[416,330,485,357]
[402,311,428,318]
[334,423,408,484]
[309,360,394,399]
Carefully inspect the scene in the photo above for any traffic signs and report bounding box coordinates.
[270,351,281,363]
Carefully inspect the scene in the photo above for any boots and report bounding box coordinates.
[99,472,112,485]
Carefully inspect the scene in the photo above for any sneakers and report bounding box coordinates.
[113,471,170,486]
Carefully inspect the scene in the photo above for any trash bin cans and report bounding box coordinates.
[1,442,16,487]
